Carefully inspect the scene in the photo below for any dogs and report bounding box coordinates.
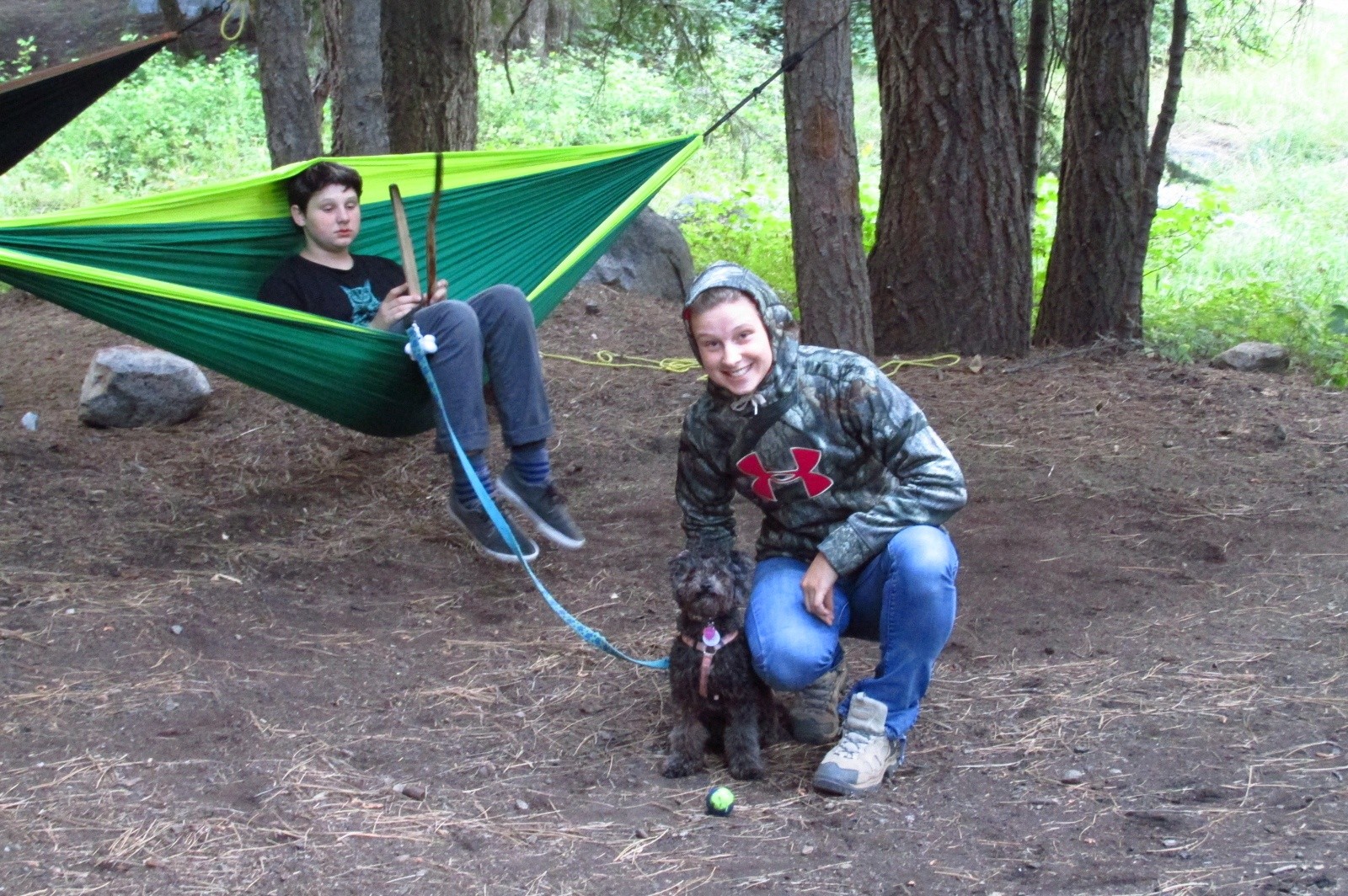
[656,537,786,778]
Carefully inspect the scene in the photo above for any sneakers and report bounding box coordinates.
[496,463,588,550]
[448,486,541,564]
[813,690,902,795]
[788,666,844,741]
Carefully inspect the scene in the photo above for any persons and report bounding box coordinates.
[674,260,967,792]
[256,161,586,563]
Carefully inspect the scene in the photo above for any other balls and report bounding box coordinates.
[705,785,736,816]
